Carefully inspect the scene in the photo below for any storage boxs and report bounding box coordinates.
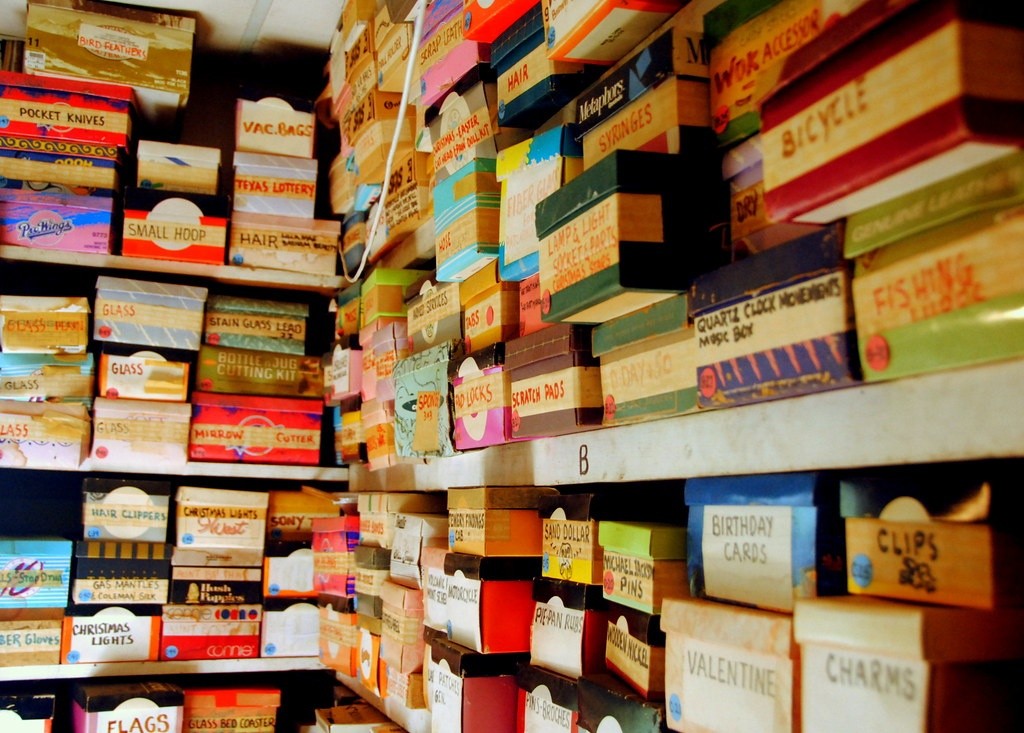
[0,0,1024,733]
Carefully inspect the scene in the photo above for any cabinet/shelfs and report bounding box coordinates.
[336,0,1023,733]
[1,243,357,682]
